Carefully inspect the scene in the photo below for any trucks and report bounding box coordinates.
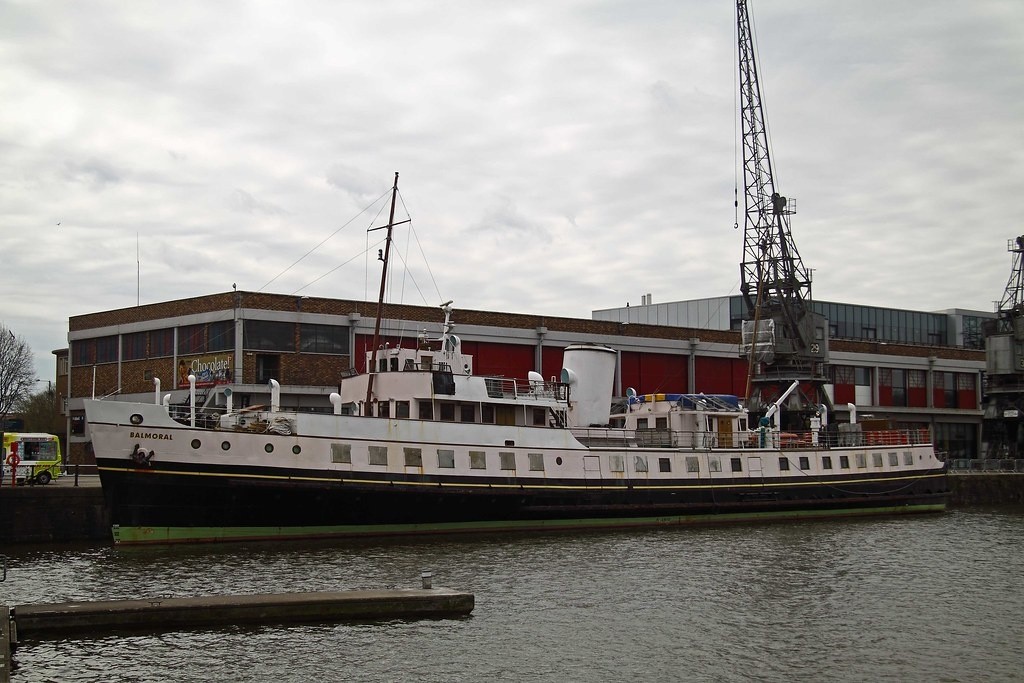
[0,431,63,487]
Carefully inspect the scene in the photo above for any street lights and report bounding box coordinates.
[36,378,51,410]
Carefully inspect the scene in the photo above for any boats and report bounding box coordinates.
[83,348,947,548]
